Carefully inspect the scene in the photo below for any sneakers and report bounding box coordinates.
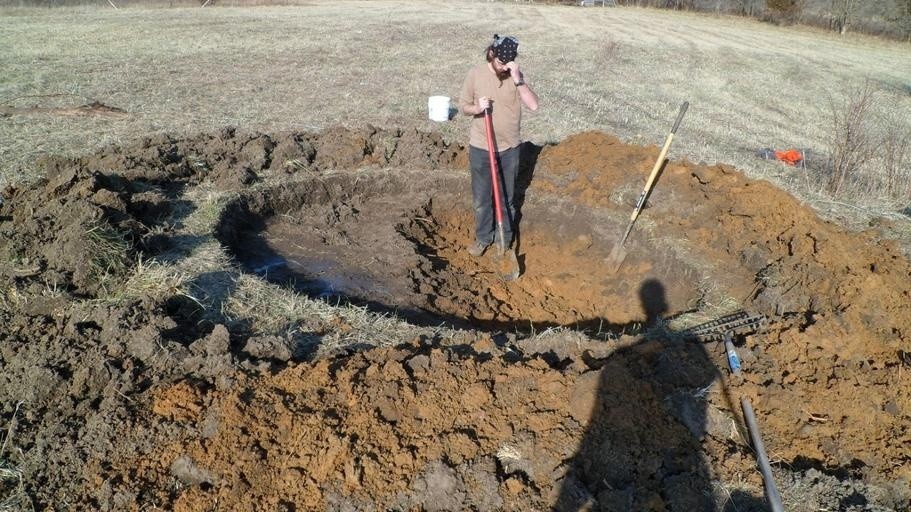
[467,239,494,257]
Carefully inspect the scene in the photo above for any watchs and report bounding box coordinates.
[514,76,525,87]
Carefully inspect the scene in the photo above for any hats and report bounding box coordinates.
[493,34,519,63]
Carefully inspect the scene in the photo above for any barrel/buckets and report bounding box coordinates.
[428,96,451,122]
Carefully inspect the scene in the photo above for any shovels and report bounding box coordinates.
[485,109,521,280]
[604,101,689,275]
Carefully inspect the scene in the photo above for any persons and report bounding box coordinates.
[458,33,538,257]
